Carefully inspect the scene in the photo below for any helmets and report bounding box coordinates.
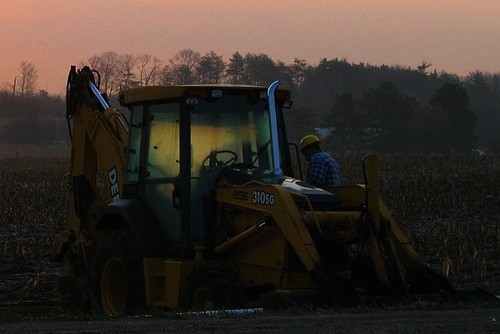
[300,135,320,151]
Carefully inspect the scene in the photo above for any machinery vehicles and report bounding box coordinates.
[60,65,460,318]
